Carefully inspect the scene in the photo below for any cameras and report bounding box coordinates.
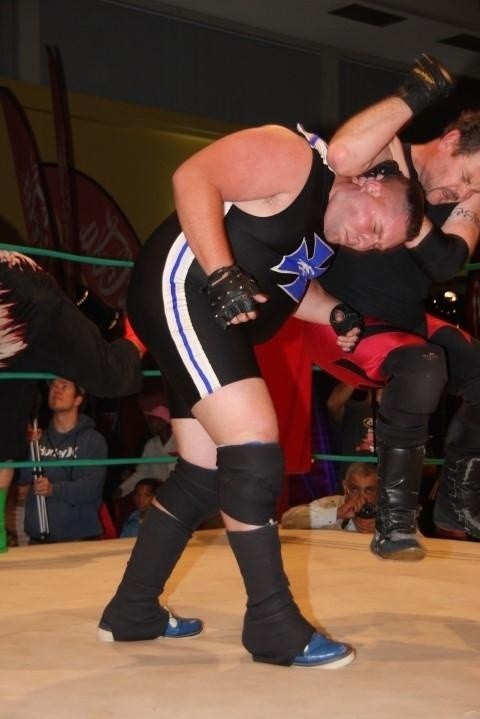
[354,503,377,519]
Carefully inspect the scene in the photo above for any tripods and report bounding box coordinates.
[28,417,49,541]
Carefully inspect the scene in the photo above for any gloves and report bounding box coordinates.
[329,303,369,336]
[207,263,262,322]
[363,159,404,178]
[392,51,455,109]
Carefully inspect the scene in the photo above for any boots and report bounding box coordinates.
[96,500,205,642]
[225,520,357,671]
[432,453,480,542]
[369,444,427,562]
